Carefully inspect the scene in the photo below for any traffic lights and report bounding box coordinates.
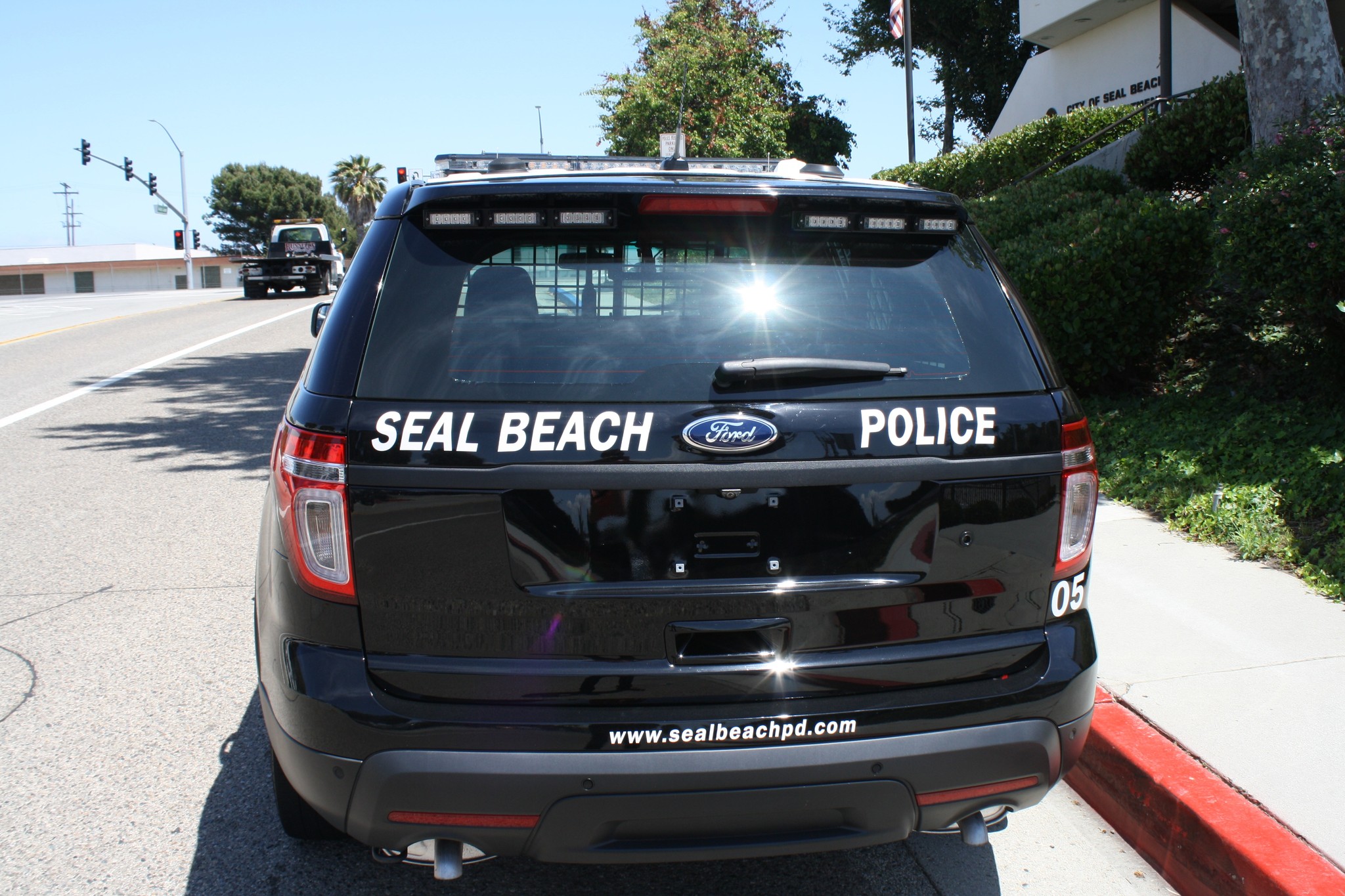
[174,230,183,249]
[193,229,200,249]
[82,139,91,165]
[397,166,408,183]
[149,173,157,195]
[124,156,134,180]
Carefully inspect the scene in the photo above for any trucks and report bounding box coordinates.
[235,218,341,296]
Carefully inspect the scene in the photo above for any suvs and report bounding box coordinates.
[258,168,1100,884]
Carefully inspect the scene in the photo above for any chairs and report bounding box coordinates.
[434,267,571,404]
